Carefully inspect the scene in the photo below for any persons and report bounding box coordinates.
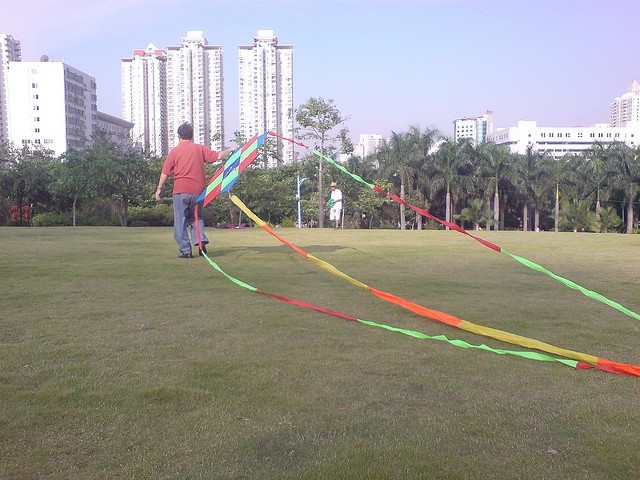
[330,182,342,230]
[156,122,237,258]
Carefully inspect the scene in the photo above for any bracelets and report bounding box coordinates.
[157,185,166,189]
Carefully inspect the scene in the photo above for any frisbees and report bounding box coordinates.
[328,199,336,206]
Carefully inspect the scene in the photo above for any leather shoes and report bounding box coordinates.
[199,249,207,256]
[178,253,192,258]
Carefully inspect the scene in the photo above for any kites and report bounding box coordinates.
[195,130,271,208]
